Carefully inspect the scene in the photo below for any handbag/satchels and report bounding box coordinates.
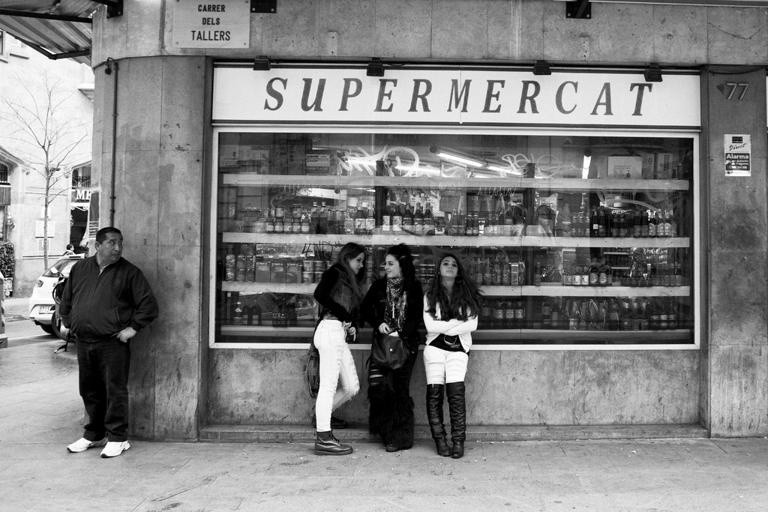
[302,343,320,399]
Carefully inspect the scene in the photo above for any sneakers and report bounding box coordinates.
[65,437,108,453]
[99,440,132,458]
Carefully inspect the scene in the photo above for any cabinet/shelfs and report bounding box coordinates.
[220,162,694,345]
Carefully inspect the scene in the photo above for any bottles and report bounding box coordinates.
[619,212,627,237]
[290,219,299,234]
[674,301,683,320]
[485,257,491,285]
[251,299,261,327]
[599,206,605,237]
[543,320,551,329]
[403,204,413,234]
[301,220,308,233]
[559,298,608,331]
[271,299,296,325]
[423,203,435,235]
[523,320,534,329]
[654,262,684,286]
[629,301,640,331]
[503,254,511,285]
[621,298,630,330]
[276,206,285,219]
[311,202,319,234]
[668,299,676,320]
[383,193,390,234]
[542,303,551,318]
[533,262,541,286]
[550,305,559,321]
[682,305,688,321]
[649,303,660,321]
[412,256,437,285]
[534,318,543,329]
[662,301,668,322]
[264,218,274,234]
[414,201,423,235]
[656,208,664,238]
[392,196,406,234]
[476,258,483,286]
[639,212,648,237]
[608,299,619,331]
[435,201,525,235]
[561,215,590,237]
[318,202,327,234]
[274,218,284,233]
[647,209,655,237]
[495,300,506,330]
[633,209,640,236]
[354,206,366,234]
[503,302,514,328]
[562,255,614,286]
[612,213,617,237]
[242,302,252,325]
[491,253,502,285]
[592,210,599,237]
[638,302,648,330]
[292,204,301,218]
[516,302,524,328]
[551,321,559,329]
[481,301,491,330]
[665,211,672,237]
[282,218,292,234]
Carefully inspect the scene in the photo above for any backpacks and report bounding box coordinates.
[370,330,408,370]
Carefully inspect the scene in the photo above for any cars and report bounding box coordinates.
[29,253,84,335]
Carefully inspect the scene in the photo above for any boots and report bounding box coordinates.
[444,381,467,459]
[425,383,452,457]
[311,405,349,429]
[400,391,417,454]
[314,429,353,456]
[378,395,404,452]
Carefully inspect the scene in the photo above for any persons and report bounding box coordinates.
[423,253,485,458]
[363,243,423,452]
[63,244,75,255]
[59,226,159,459]
[312,243,368,456]
